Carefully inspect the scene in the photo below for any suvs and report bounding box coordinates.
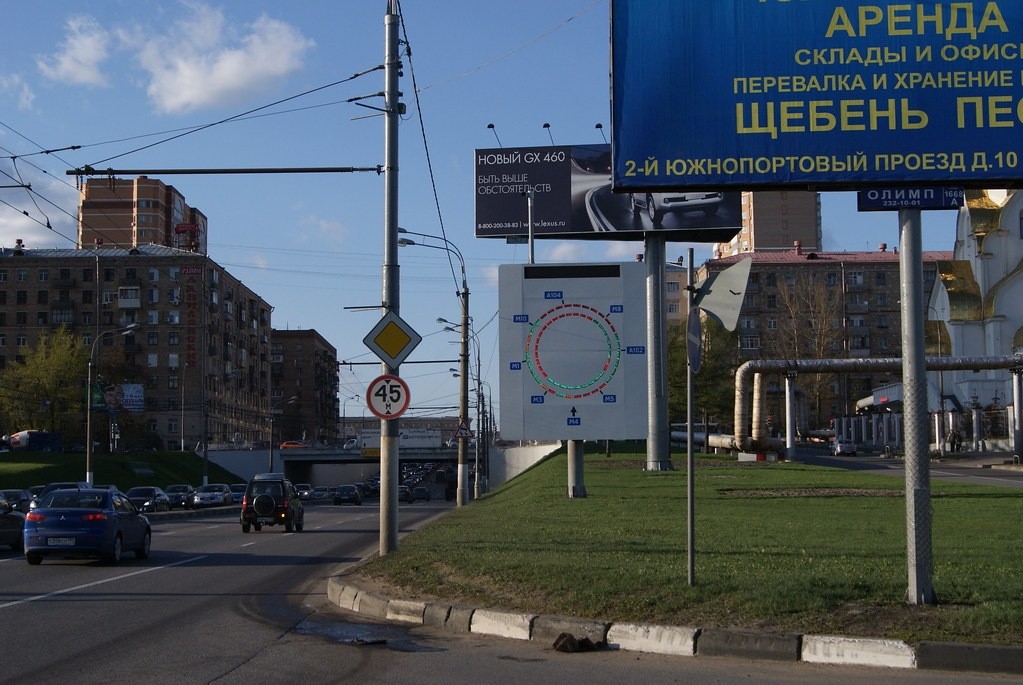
[240,473,304,532]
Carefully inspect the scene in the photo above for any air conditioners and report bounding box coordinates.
[163,317,170,322]
[172,301,178,305]
[168,366,175,370]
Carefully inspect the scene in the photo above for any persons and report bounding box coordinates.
[104,385,128,413]
[947,429,962,453]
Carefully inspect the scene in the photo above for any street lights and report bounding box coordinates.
[86,323,140,485]
[181,363,189,451]
[436,318,480,499]
[398,227,470,508]
[269,394,297,473]
[450,368,492,436]
[343,394,359,438]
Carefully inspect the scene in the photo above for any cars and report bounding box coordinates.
[436,462,475,501]
[0,482,151,565]
[293,463,438,505]
[230,484,247,503]
[280,441,333,449]
[193,484,234,506]
[631,190,723,223]
[831,438,856,456]
[126,487,170,513]
[165,485,197,508]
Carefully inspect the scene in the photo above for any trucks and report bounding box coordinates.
[343,432,361,450]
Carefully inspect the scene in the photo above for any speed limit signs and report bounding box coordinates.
[366,374,411,420]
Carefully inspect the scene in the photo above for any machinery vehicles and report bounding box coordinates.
[10,430,64,453]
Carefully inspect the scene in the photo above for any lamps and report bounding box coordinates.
[595,123,607,143]
[487,124,502,148]
[543,123,555,146]
